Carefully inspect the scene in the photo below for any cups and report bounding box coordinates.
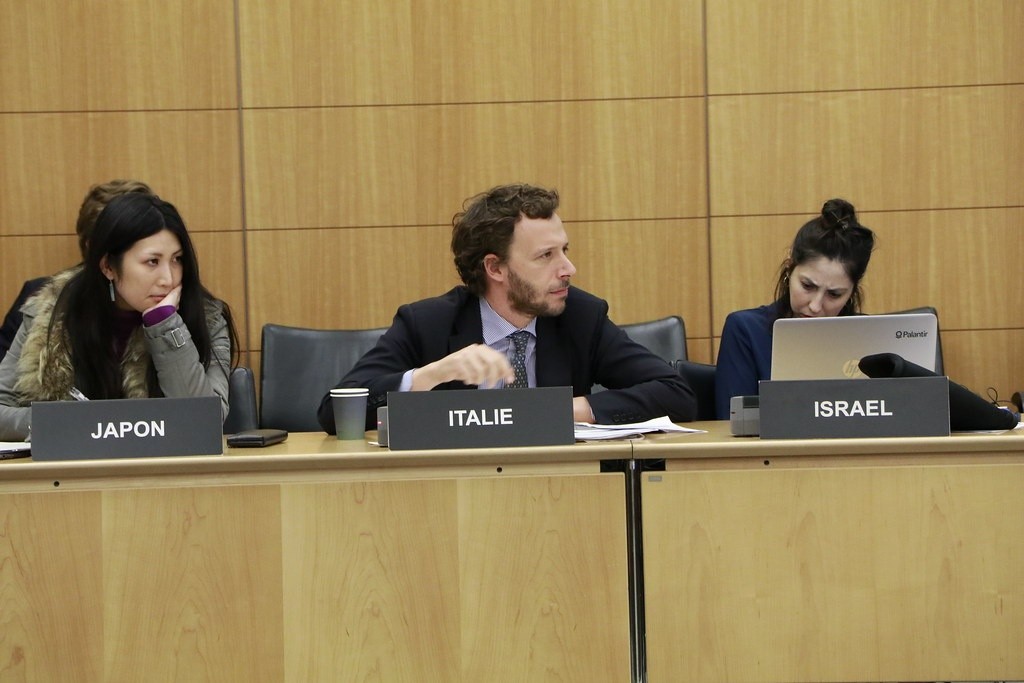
[330,394,369,440]
[330,388,369,395]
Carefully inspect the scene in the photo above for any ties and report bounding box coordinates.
[505,331,531,389]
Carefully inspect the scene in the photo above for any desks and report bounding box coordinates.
[1,411,1024,682]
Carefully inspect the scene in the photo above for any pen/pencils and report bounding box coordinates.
[68,387,90,401]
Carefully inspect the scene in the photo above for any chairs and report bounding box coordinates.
[621,317,717,418]
[262,322,390,431]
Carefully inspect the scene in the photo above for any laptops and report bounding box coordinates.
[771,313,937,381]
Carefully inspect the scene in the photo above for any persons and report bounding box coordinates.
[714,198,876,422]
[0,193,242,442]
[315,182,699,434]
[0,179,153,367]
